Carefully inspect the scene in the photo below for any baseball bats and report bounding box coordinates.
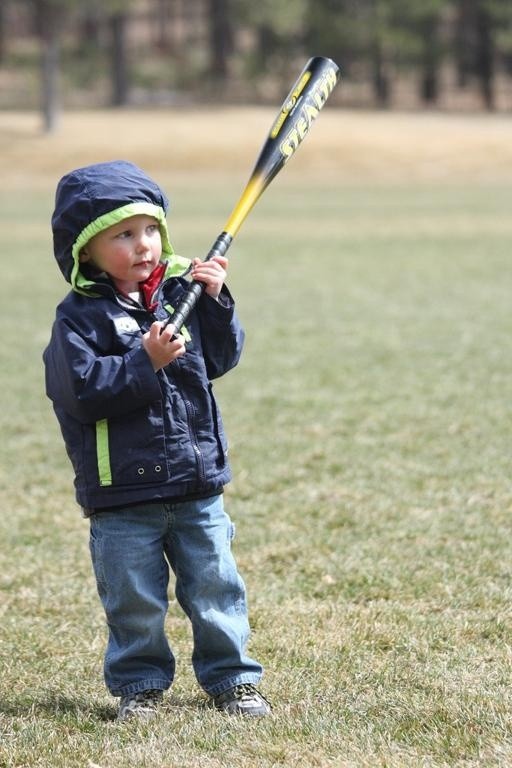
[159,56,342,344]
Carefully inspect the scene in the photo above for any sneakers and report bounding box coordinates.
[211,685,271,720]
[116,689,162,725]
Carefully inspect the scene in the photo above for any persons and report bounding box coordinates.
[42,160,273,724]
